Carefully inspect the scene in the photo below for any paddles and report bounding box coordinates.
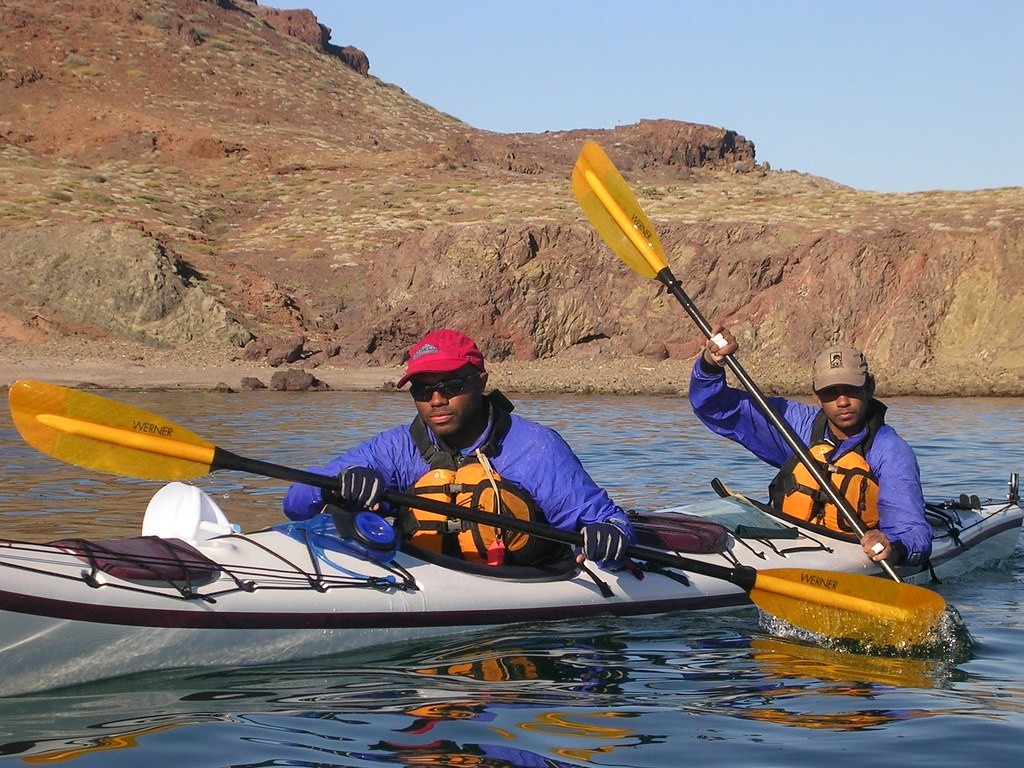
[5,378,948,647]
[569,133,905,586]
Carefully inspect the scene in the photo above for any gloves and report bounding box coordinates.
[573,520,630,565]
[321,465,386,511]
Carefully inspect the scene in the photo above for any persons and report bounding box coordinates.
[283,328,637,570]
[688,324,933,567]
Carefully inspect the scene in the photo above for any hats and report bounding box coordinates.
[397,330,486,388]
[812,345,869,392]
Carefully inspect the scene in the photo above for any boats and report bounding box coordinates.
[0,469,1023,683]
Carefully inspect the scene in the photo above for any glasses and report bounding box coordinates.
[409,372,481,402]
